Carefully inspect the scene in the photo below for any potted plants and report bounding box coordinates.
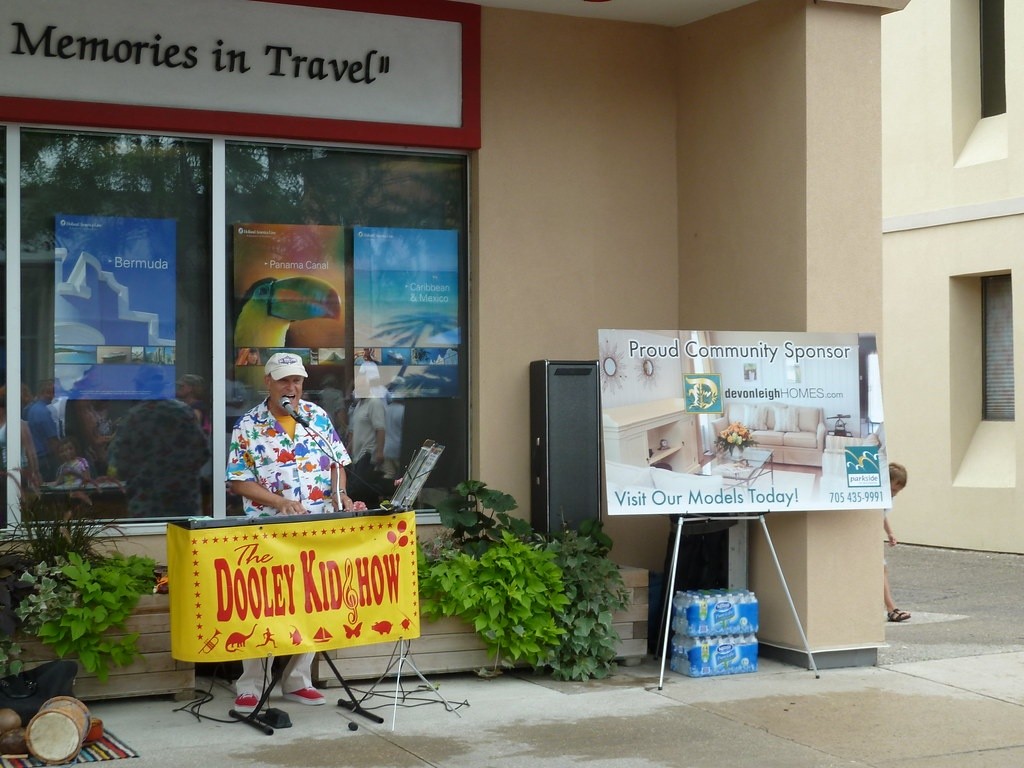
[311,481,650,691]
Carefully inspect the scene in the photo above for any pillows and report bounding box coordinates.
[774,408,801,433]
[744,403,768,431]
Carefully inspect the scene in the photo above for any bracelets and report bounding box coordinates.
[332,488,346,496]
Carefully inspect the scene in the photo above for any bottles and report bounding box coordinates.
[669,587,759,678]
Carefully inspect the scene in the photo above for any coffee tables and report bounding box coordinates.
[694,445,774,501]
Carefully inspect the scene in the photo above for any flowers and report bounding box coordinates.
[714,421,759,456]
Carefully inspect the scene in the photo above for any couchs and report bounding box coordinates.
[710,401,825,466]
[820,421,891,507]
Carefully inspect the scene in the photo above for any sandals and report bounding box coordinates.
[887,607,911,622]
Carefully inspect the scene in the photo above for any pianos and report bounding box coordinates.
[170,506,444,532]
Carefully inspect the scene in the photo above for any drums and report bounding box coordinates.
[26,696,92,764]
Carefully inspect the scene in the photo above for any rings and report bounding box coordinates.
[347,500,350,502]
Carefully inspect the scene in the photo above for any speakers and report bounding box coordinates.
[529,360,601,545]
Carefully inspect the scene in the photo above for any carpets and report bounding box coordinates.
[713,468,816,499]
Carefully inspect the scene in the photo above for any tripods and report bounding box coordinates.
[350,449,462,730]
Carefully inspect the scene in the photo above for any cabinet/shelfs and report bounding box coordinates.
[602,399,701,475]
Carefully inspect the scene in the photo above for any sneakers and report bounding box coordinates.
[234,692,259,713]
[282,685,325,705]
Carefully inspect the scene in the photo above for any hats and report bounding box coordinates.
[132,364,165,385]
[264,352,308,380]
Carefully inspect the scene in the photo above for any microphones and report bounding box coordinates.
[281,397,301,424]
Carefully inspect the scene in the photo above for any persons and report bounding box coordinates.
[226,354,355,714]
[884,462,911,622]
[1,356,406,519]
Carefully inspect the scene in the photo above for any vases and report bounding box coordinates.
[730,447,744,461]
